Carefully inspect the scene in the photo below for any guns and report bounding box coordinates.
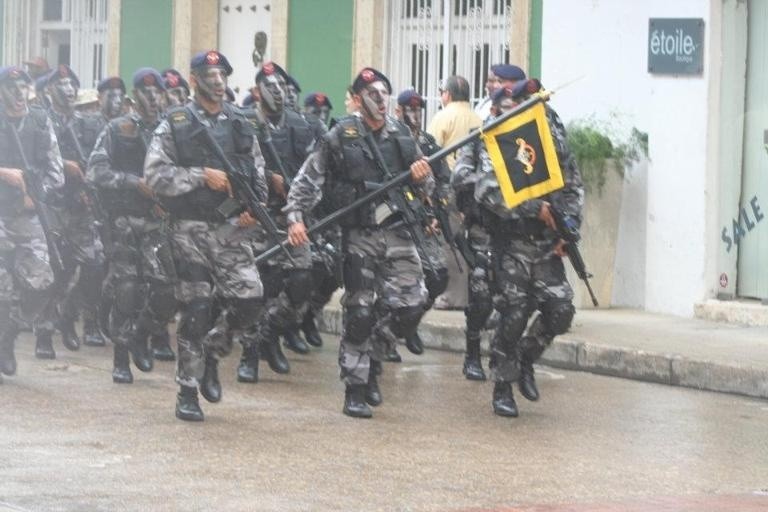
[407,182,445,248]
[132,131,172,220]
[534,191,599,305]
[257,123,335,282]
[432,177,465,275]
[9,123,65,273]
[191,127,296,269]
[64,119,104,214]
[363,133,442,281]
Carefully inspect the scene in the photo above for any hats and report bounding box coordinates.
[0,57,80,89]
[399,90,424,107]
[99,77,125,92]
[243,63,298,106]
[489,63,542,102]
[305,95,332,109]
[352,67,392,94]
[133,66,188,91]
[190,52,233,75]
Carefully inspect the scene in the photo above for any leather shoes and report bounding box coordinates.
[0,339,15,375]
[284,317,322,352]
[84,327,103,344]
[342,360,383,420]
[200,357,225,403]
[520,364,539,401]
[492,383,518,417]
[388,333,424,363]
[175,386,204,421]
[54,319,78,351]
[35,334,52,355]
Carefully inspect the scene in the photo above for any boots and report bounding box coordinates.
[111,334,174,383]
[462,336,485,380]
[238,337,288,381]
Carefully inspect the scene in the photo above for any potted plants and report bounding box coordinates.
[561,111,644,309]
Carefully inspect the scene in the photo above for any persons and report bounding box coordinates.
[284,65,584,417]
[0,52,335,424]
